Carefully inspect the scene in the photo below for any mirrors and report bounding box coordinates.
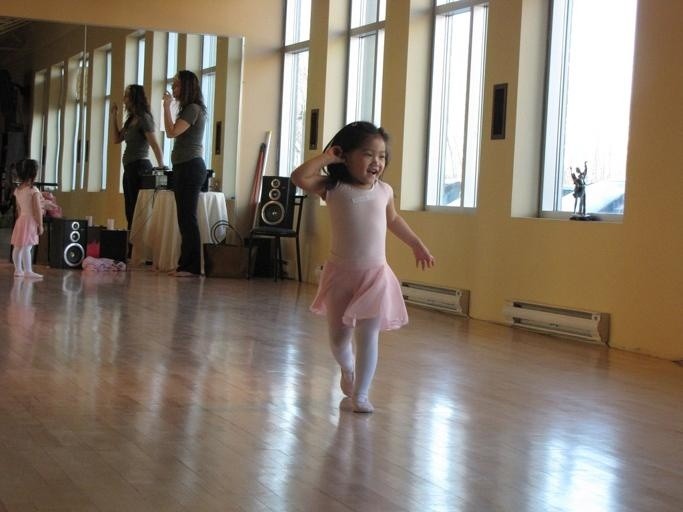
[0,17,245,271]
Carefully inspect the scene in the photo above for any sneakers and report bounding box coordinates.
[24,272,44,278]
[14,271,25,276]
[340,360,355,397]
[353,396,375,412]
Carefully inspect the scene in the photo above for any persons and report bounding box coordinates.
[571,159,588,216]
[13,160,45,278]
[163,71,207,277]
[111,85,164,260]
[291,122,435,413]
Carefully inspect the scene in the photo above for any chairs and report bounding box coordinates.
[244,195,308,284]
[8,182,60,265]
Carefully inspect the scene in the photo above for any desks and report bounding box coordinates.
[143,189,228,271]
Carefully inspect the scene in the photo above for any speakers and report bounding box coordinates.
[259,175,296,230]
[88,225,107,244]
[100,229,132,262]
[50,219,88,270]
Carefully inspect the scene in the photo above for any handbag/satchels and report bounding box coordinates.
[203,243,248,280]
